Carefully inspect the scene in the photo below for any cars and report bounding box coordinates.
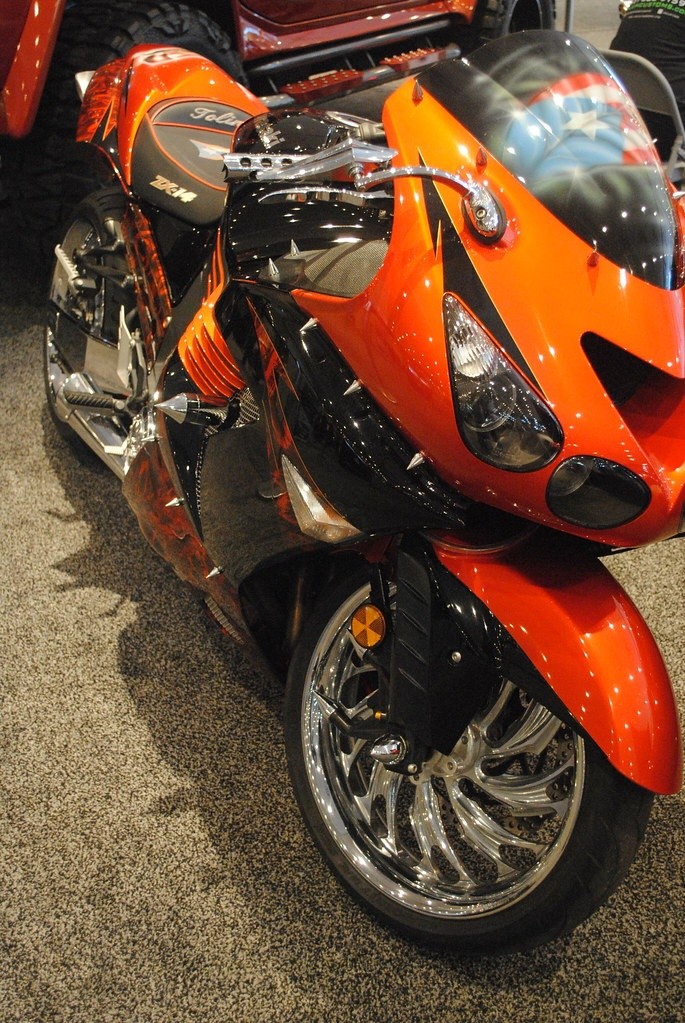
[0,2,568,304]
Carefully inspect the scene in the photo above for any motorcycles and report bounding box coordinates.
[43,30,685,960]
